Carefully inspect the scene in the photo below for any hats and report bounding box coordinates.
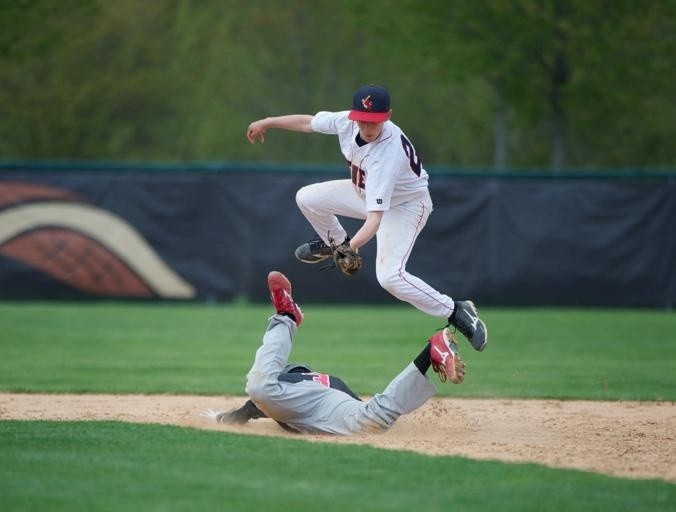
[348,84,391,122]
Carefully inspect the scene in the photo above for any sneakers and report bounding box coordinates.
[267,271,303,327]
[448,300,487,352]
[295,237,351,264]
[426,328,466,384]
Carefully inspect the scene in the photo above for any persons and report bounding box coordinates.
[199,270,466,439]
[246,83,489,353]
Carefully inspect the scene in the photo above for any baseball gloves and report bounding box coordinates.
[316,230,362,277]
